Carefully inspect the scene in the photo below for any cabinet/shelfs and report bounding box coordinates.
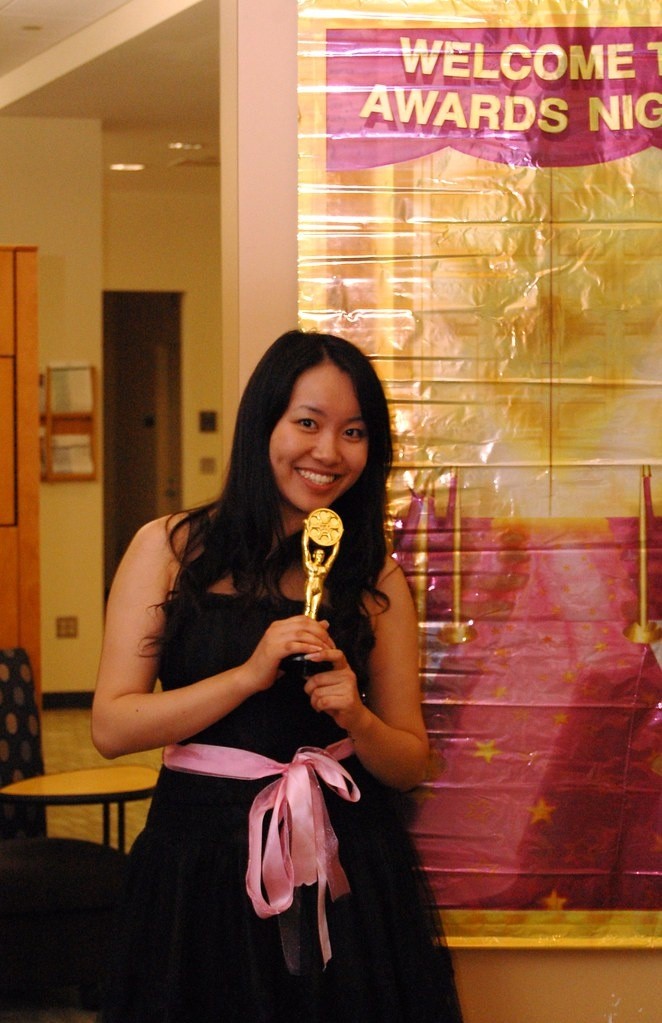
[0,243,40,726]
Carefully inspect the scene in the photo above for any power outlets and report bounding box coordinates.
[57,617,78,639]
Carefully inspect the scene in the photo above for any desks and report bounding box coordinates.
[0,765,162,856]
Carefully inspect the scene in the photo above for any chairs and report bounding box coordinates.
[0,646,127,1015]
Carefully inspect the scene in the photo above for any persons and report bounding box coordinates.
[92,329,466,1023]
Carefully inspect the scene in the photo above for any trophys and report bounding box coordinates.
[276,508,344,676]
